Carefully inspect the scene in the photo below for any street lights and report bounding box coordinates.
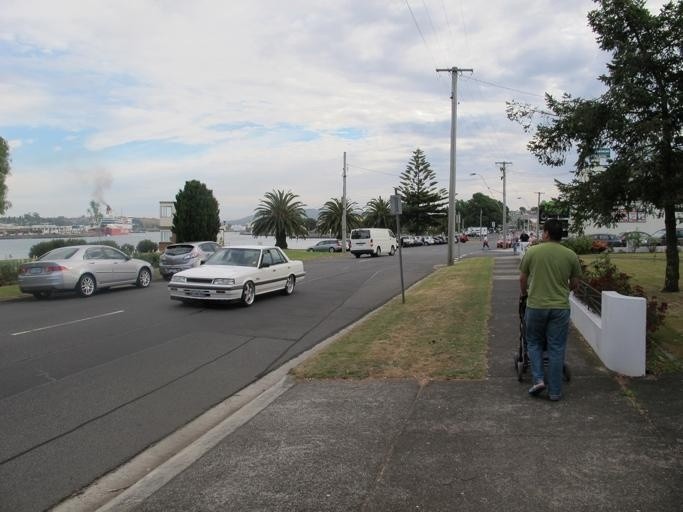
[469,172,507,248]
[516,195,530,211]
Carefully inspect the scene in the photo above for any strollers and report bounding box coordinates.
[512,290,573,383]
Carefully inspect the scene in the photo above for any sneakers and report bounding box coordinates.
[528,383,545,394]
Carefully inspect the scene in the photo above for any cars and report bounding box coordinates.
[157,240,224,282]
[617,231,654,247]
[494,225,523,248]
[397,233,473,245]
[588,232,623,247]
[166,243,308,310]
[306,237,350,253]
[14,242,158,300]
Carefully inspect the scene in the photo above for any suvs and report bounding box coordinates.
[640,226,682,246]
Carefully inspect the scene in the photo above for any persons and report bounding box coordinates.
[482,234,491,250]
[519,219,583,401]
[511,230,536,255]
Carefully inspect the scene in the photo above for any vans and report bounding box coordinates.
[347,226,398,259]
